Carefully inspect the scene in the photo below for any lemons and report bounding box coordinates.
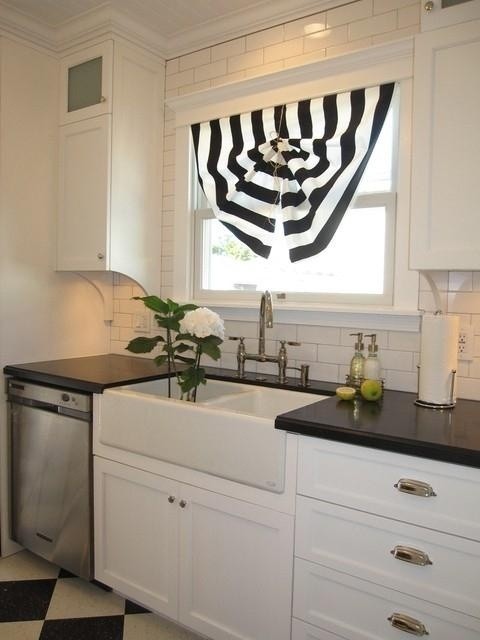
[336,386,356,400]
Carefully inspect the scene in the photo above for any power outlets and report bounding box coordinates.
[457,327,474,360]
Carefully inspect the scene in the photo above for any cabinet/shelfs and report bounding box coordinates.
[407,0,480,313]
[292,435,480,640]
[92,457,296,640]
[53,39,166,323]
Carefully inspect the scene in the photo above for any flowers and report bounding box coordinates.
[124,295,225,402]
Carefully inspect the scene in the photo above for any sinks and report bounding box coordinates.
[100,370,258,471]
[205,386,340,498]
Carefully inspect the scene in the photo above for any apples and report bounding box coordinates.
[360,380,383,400]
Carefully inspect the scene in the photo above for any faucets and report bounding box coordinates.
[258,291,275,356]
